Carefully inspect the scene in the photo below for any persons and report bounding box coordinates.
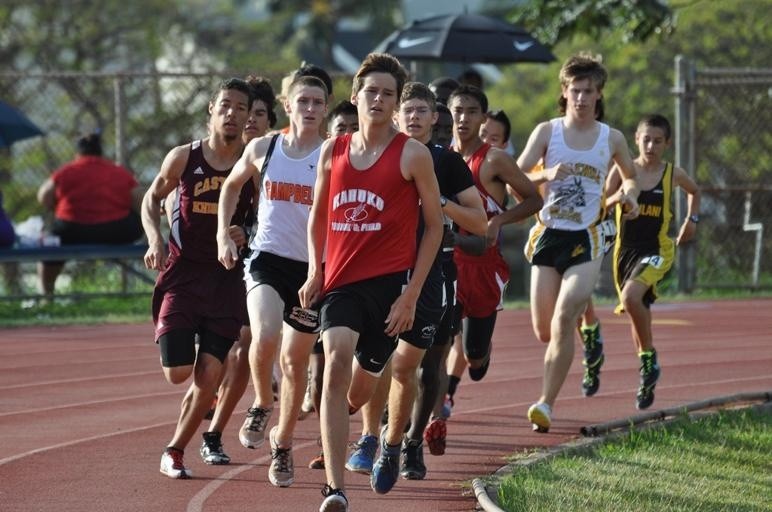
[392,99,489,480]
[450,85,542,443]
[262,65,333,137]
[442,111,511,419]
[141,78,254,477]
[297,56,443,512]
[311,97,360,469]
[217,76,324,487]
[38,134,146,303]
[382,77,459,434]
[273,69,328,413]
[505,55,640,432]
[603,113,702,410]
[0,162,25,297]
[199,76,274,463]
[514,83,633,396]
[346,81,488,493]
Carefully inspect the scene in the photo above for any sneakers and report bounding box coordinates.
[346,435,377,472]
[269,425,295,486]
[300,366,315,412]
[425,420,446,456]
[198,431,231,466]
[579,323,607,397]
[400,435,427,481]
[371,424,402,495]
[319,484,348,512]
[636,350,660,409]
[527,402,552,433]
[159,448,193,480]
[436,396,454,422]
[469,340,492,381]
[203,392,218,419]
[240,404,274,450]
[309,452,324,468]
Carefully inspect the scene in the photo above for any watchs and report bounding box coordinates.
[243,224,251,238]
[684,214,699,223]
[441,194,447,207]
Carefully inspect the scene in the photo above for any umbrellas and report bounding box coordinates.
[370,4,559,84]
[0,98,44,148]
